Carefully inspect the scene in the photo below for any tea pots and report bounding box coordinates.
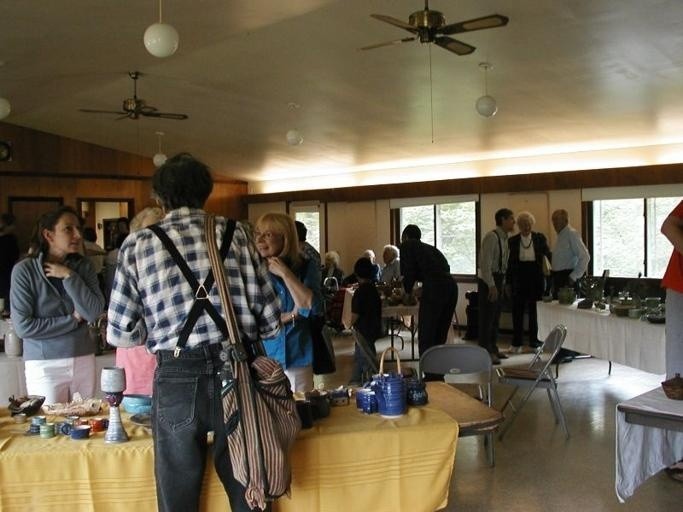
[370,346,408,420]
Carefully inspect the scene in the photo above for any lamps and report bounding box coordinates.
[142,0,180,58]
[285,102,303,147]
[475,62,500,117]
[0,98,11,120]
[152,132,168,167]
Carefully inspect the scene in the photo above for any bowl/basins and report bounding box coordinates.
[123,394,152,413]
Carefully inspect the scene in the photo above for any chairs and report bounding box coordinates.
[496,324,572,445]
[418,343,496,470]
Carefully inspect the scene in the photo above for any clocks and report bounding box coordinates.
[0,142,11,162]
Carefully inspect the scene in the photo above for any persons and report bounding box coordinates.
[399,223,459,382]
[10,207,106,403]
[82,226,107,274]
[110,217,130,249]
[508,211,552,347]
[349,257,383,386]
[247,212,325,394]
[319,250,345,287]
[380,244,401,285]
[115,207,166,394]
[476,208,515,365]
[548,208,590,299]
[342,249,379,284]
[294,221,321,268]
[108,153,284,510]
[661,200,682,484]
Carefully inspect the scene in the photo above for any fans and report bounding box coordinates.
[80,70,188,122]
[354,1,509,58]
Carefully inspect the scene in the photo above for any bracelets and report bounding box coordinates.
[290,309,296,326]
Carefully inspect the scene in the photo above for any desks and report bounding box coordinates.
[334,284,420,364]
[536,299,666,378]
[0,379,501,511]
[615,375,683,433]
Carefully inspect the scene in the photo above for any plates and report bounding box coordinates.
[129,411,155,427]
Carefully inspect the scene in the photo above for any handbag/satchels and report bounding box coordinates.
[206,212,301,509]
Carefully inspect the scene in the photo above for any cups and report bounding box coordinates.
[371,373,389,381]
[355,388,372,409]
[39,423,54,439]
[362,391,378,414]
[32,416,46,427]
